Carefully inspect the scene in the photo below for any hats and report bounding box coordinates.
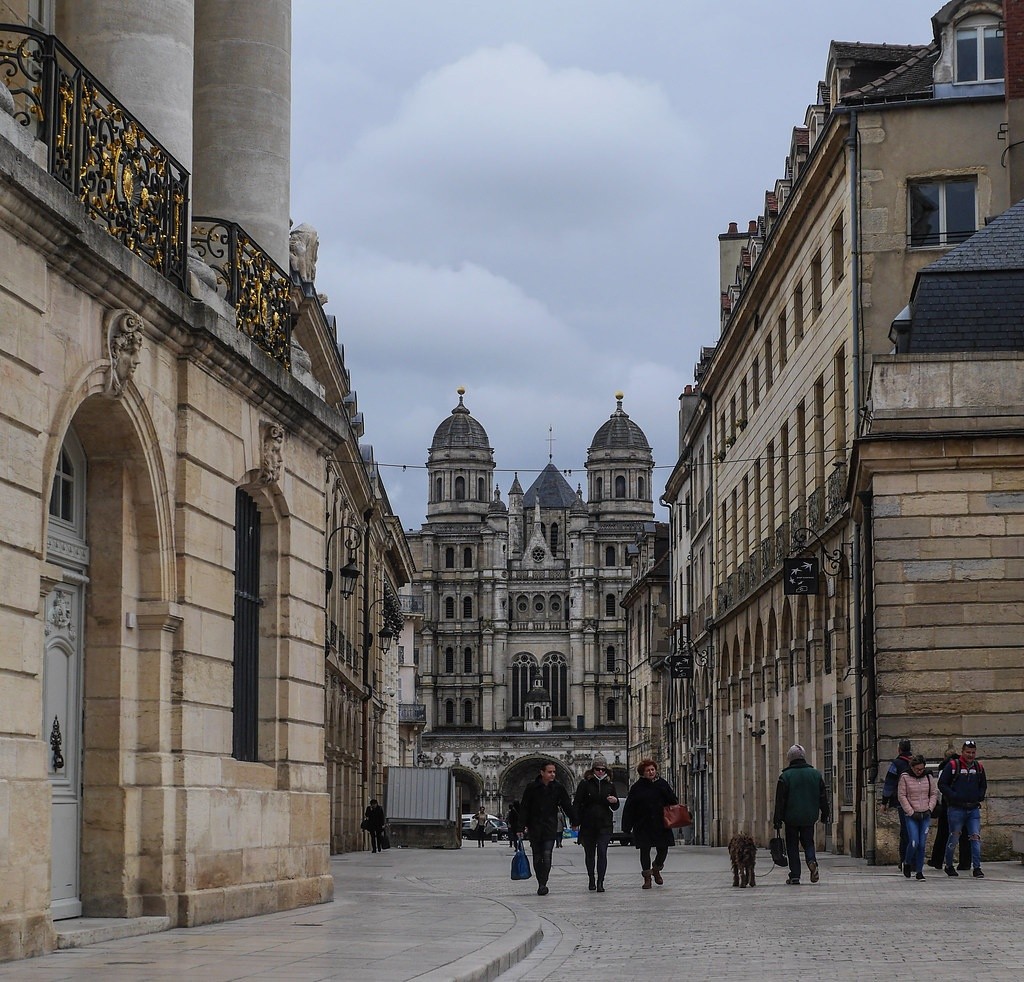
[787,744,805,762]
[592,753,607,768]
[899,738,911,752]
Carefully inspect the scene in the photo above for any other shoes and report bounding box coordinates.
[898,864,901,869]
[973,868,984,878]
[537,886,548,895]
[589,877,596,890]
[597,881,605,892]
[808,861,819,883]
[786,878,800,884]
[928,860,942,869]
[945,866,958,876]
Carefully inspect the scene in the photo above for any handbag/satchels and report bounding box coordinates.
[664,803,691,828]
[380,829,390,849]
[361,818,369,830]
[511,838,531,880]
[770,829,788,866]
[930,803,941,818]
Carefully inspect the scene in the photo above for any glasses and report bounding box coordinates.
[966,741,976,745]
[595,767,607,772]
[913,766,925,772]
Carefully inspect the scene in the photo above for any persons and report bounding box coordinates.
[572,753,620,892]
[365,799,385,853]
[928,748,972,870]
[516,762,576,895]
[505,800,528,851]
[556,806,567,848]
[620,759,692,889]
[773,744,830,884]
[898,755,937,881]
[882,739,916,877]
[472,806,488,848]
[937,740,987,878]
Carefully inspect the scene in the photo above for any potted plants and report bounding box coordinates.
[735,417,747,432]
[712,450,726,464]
[723,436,736,448]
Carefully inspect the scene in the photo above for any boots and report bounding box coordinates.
[653,870,663,885]
[642,870,652,889]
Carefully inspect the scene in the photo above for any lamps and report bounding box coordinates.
[325,526,363,599]
[611,658,631,703]
[369,600,393,654]
[384,686,396,696]
[751,726,767,738]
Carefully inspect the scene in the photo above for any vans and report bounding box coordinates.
[612,797,629,847]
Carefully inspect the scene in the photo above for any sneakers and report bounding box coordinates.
[902,862,911,878]
[916,873,926,882]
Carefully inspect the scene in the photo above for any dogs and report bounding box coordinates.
[728,833,756,888]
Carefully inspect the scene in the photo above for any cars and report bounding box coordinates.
[461,814,508,838]
